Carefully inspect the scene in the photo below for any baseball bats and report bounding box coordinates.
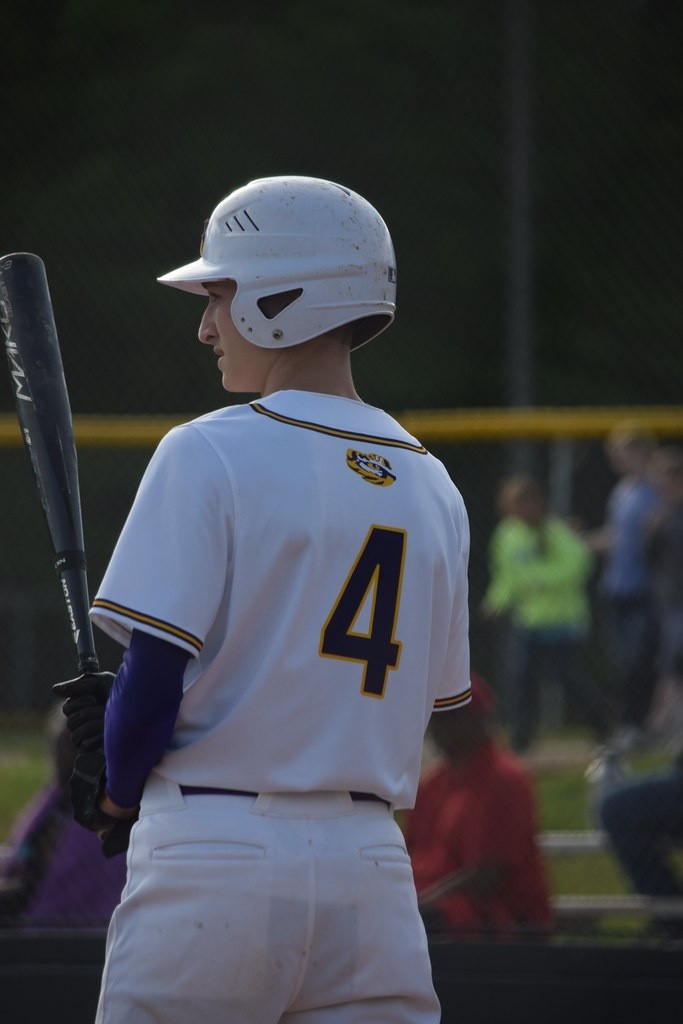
[1,250,100,677]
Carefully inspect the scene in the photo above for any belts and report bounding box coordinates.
[178,783,392,810]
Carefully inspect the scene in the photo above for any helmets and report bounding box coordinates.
[157,175,397,353]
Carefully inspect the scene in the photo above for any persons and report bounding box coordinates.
[0,698,126,932]
[404,673,556,933]
[54,174,475,1024]
[584,422,682,940]
[478,469,613,752]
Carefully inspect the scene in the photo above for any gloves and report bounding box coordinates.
[66,745,142,836]
[51,671,117,756]
